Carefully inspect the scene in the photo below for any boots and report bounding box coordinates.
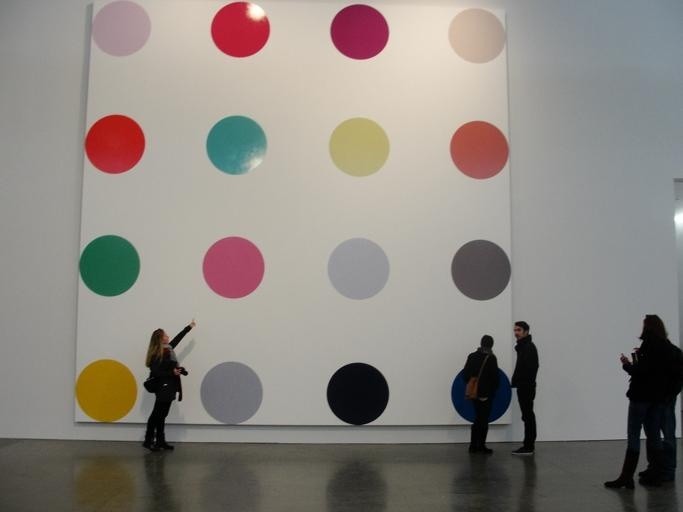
[143,431,174,451]
[639,463,675,485]
[605,477,634,489]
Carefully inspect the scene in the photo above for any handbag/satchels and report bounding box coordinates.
[144,376,171,393]
[466,377,479,399]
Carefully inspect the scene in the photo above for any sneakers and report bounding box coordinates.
[512,447,535,455]
[469,446,493,454]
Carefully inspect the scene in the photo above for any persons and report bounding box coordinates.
[463,321,540,457]
[604,314,683,490]
[142,316,196,452]
